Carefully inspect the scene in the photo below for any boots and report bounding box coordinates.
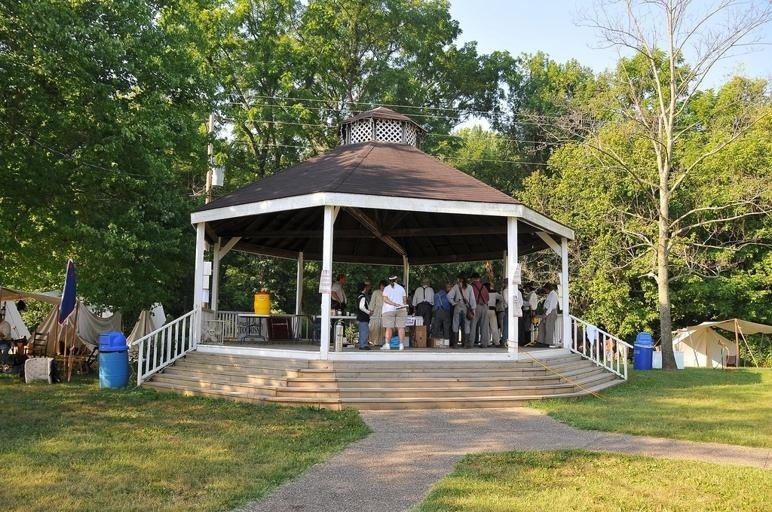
[461,332,470,349]
[449,332,459,349]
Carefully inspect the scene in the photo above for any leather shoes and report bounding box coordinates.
[478,344,506,348]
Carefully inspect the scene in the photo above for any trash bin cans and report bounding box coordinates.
[98,330,129,388]
[634,332,654,370]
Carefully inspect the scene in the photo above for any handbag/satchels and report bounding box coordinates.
[466,307,474,320]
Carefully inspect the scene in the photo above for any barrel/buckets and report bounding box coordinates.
[253,291,274,317]
[98,352,130,391]
[632,344,654,370]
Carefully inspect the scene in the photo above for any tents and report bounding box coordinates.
[668,322,737,367]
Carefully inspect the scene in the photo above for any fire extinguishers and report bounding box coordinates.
[333,318,345,352]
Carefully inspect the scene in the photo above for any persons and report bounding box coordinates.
[330,273,349,348]
[354,271,560,352]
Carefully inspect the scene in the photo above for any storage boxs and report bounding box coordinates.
[409,326,427,347]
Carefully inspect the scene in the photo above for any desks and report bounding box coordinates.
[315,315,357,349]
[238,313,271,344]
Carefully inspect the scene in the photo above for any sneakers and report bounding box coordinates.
[359,343,404,352]
[532,341,549,348]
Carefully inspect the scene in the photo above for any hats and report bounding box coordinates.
[388,274,399,279]
[542,281,556,290]
[420,277,430,283]
[471,271,481,278]
[522,282,536,290]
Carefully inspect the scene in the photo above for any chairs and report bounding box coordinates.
[24,331,50,374]
